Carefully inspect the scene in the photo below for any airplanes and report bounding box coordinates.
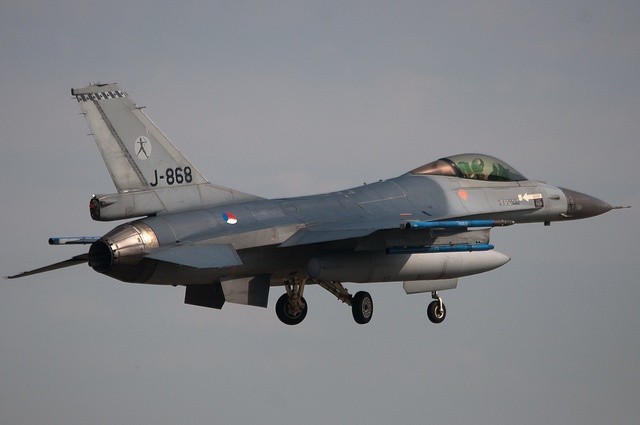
[9,76,629,324]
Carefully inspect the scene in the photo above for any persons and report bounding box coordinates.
[470,158,489,180]
